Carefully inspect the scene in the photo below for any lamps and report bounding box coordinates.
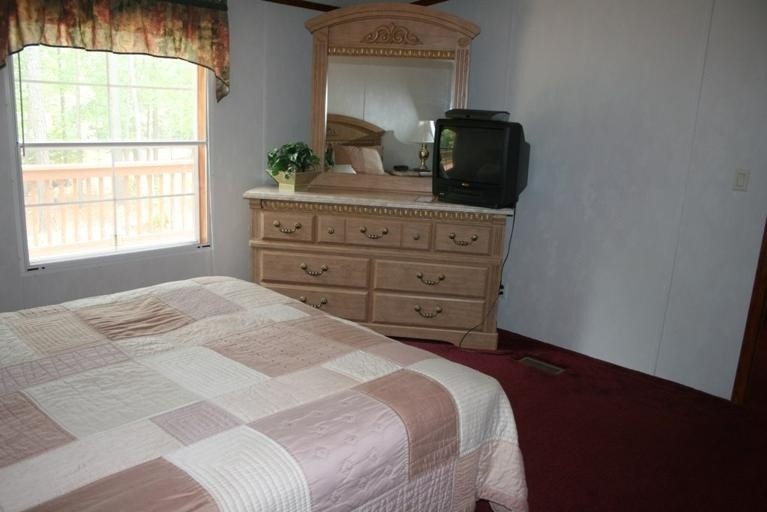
[409,120,435,171]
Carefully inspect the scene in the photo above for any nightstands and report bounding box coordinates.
[387,168,434,177]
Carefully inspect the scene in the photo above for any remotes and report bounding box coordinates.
[393,165,408,171]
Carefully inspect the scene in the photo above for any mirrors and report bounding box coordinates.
[305,4,482,197]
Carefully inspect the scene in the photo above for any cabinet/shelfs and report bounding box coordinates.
[244,186,516,351]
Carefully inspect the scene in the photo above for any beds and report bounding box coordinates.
[325,113,385,175]
[0,273,532,512]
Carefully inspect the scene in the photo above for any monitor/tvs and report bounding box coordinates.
[432,119,530,208]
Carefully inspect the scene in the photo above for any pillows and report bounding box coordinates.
[326,164,356,174]
[362,147,384,174]
[334,145,368,174]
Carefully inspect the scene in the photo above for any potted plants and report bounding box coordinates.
[266,143,320,192]
